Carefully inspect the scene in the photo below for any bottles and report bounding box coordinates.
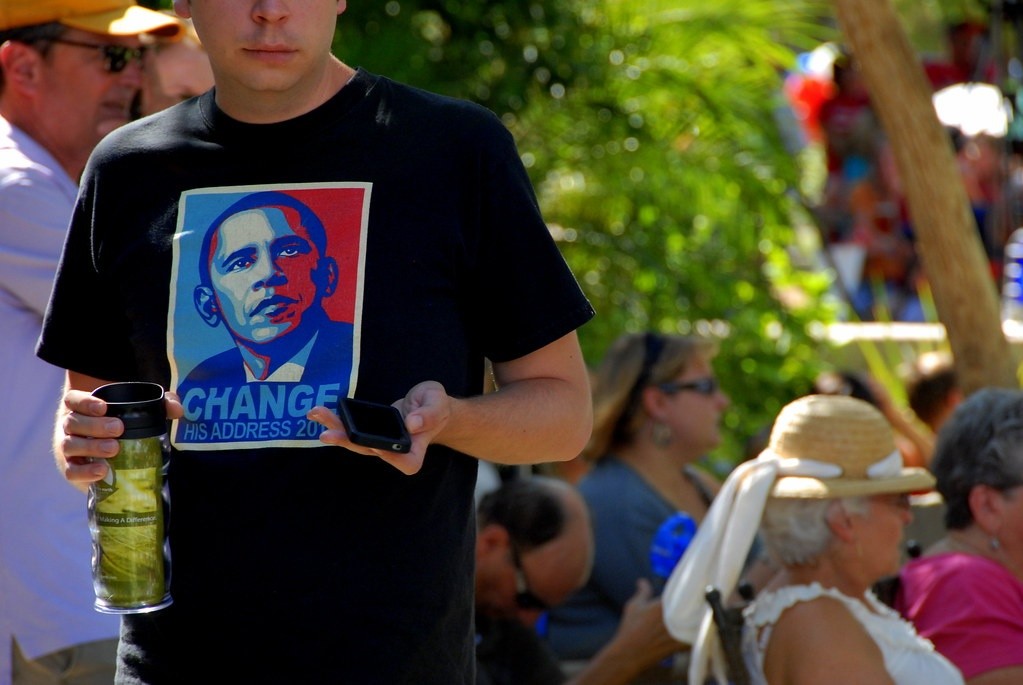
[1002,226,1023,336]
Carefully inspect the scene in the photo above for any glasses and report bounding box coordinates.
[512,552,553,621]
[665,377,717,395]
[23,35,149,74]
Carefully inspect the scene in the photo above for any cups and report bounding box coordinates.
[88,383,175,614]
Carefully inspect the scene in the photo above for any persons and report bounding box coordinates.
[0,0,215,685]
[474,332,728,685]
[754,359,1023,685]
[663,393,963,685]
[38,0,599,685]
[818,59,1023,327]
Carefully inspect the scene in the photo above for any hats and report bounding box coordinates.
[0,0,186,42]
[661,394,938,684]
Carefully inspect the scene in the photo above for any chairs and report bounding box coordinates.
[705,581,756,685]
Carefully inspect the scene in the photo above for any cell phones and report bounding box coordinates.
[338,397,414,452]
